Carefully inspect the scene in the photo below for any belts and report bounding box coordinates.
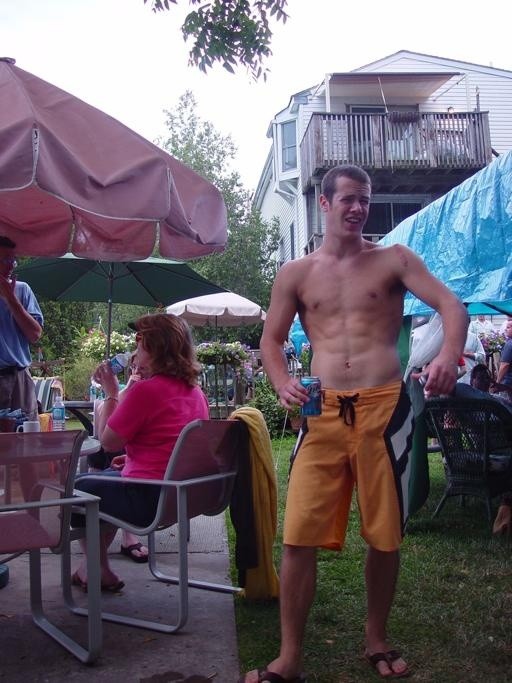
[0,367,26,376]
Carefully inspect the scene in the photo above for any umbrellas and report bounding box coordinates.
[166,289,267,342]
[0,57,226,265]
[14,252,230,361]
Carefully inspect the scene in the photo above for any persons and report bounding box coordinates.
[0,235,45,520]
[88,347,149,563]
[71,313,210,593]
[441,313,512,537]
[237,163,471,683]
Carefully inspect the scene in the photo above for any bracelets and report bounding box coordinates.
[103,396,118,404]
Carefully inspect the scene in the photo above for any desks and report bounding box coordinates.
[64,401,96,436]
[0,436,101,591]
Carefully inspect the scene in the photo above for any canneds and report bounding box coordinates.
[300,377,321,418]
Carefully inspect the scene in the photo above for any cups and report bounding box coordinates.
[499,392,508,398]
[15,421,41,446]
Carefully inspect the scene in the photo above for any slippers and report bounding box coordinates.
[236,665,308,683]
[71,571,126,594]
[360,643,412,679]
[120,541,151,564]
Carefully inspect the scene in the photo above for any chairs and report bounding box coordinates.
[470,364,492,393]
[61,418,245,635]
[0,430,102,665]
[425,396,512,531]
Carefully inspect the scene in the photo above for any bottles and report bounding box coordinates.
[36,347,43,362]
[90,351,132,388]
[51,396,66,434]
[90,384,96,402]
[98,391,105,400]
